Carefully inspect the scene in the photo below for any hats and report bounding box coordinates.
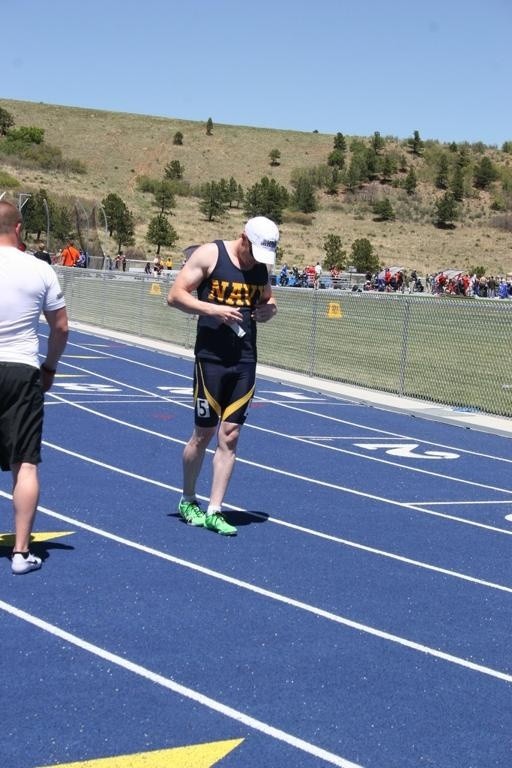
[245,216,280,265]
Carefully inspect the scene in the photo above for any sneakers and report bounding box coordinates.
[10,551,42,573]
[177,497,238,535]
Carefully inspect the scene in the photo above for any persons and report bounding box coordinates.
[269,258,512,301]
[16,237,186,295]
[0,197,70,575]
[166,215,280,537]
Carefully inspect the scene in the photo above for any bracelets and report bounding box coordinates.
[40,362,57,376]
